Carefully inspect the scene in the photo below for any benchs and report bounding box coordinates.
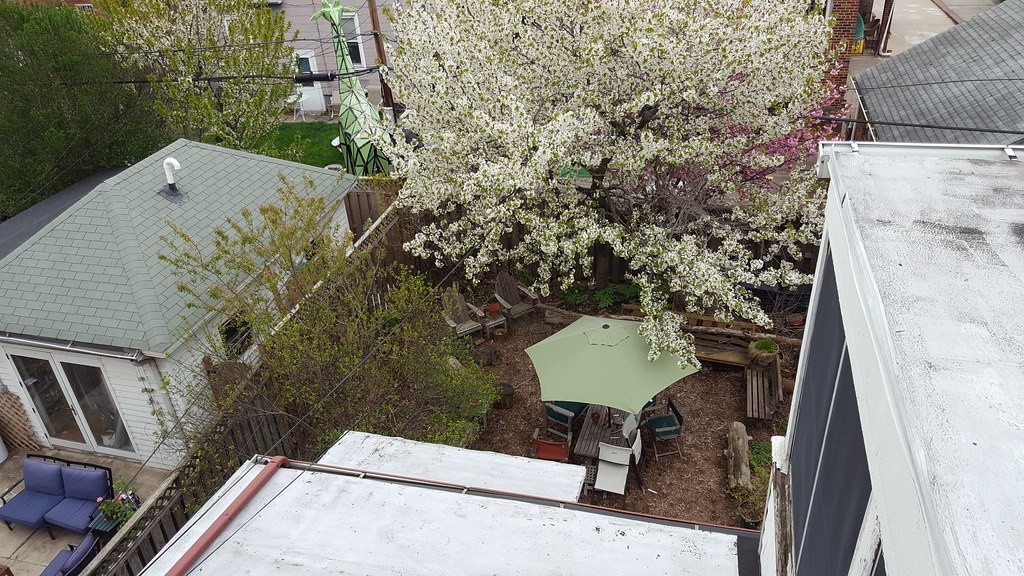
[747,355,784,421]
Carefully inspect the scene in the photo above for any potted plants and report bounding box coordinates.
[752,338,779,365]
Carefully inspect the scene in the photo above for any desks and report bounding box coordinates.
[574,404,643,464]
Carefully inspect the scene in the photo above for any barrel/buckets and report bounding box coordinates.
[102,430,115,445]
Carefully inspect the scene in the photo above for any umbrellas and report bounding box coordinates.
[523,315,701,428]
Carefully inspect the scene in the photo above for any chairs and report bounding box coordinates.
[643,394,686,461]
[543,401,590,450]
[441,288,485,338]
[594,442,632,495]
[530,428,572,464]
[495,271,538,331]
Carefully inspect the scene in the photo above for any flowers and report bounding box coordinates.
[94,494,125,518]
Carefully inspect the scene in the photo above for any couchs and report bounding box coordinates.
[39,529,100,576]
[0,453,114,540]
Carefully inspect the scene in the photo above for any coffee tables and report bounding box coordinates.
[88,511,121,553]
[481,313,506,341]
